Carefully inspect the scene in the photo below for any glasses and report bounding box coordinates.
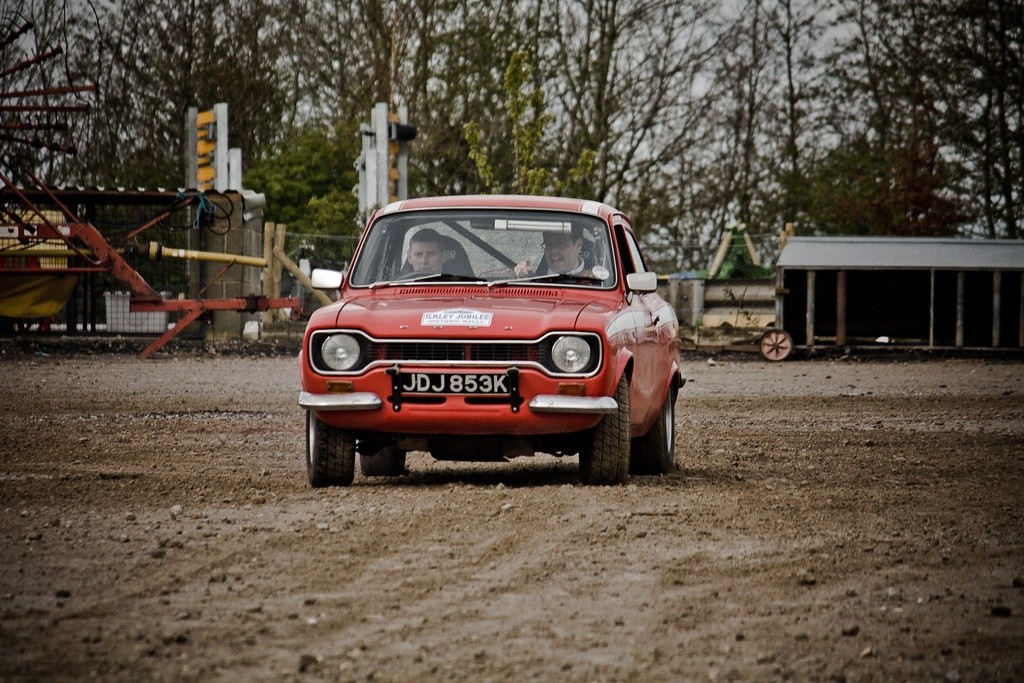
[541,238,572,250]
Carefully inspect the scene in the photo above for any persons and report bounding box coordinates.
[406,228,447,274]
[514,223,592,279]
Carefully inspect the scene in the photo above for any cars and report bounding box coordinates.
[297,193,687,489]
